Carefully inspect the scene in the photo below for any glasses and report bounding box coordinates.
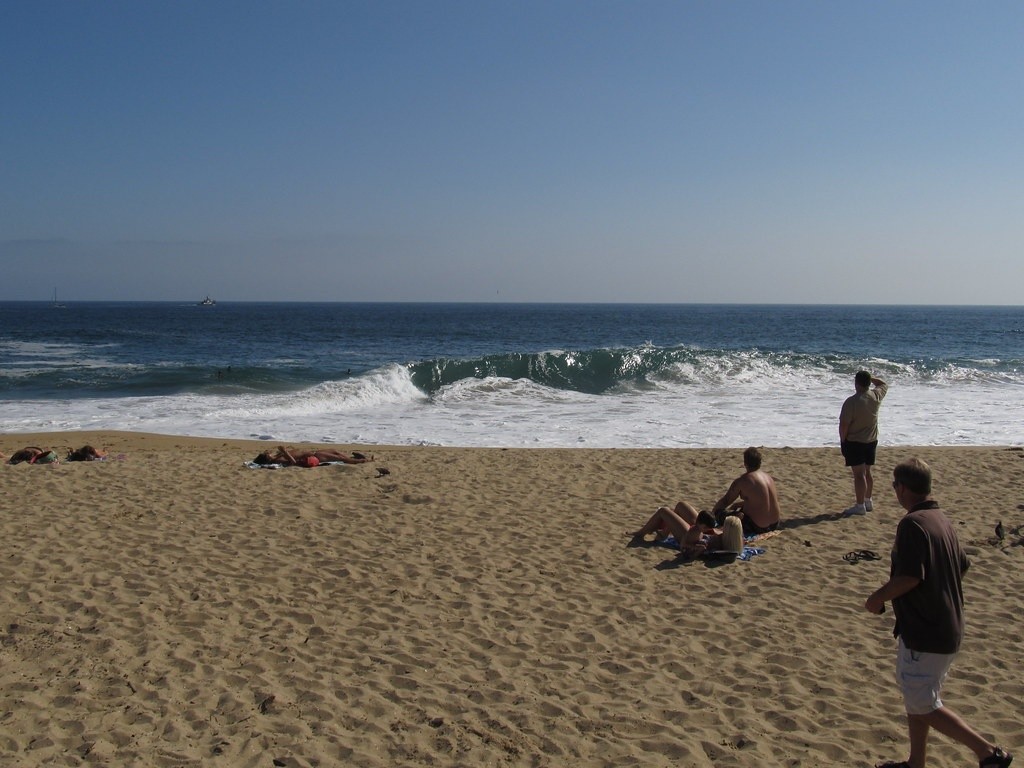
[893,481,898,489]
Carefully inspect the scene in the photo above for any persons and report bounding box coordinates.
[5,447,108,464]
[633,501,744,556]
[839,371,889,516]
[254,446,374,463]
[866,456,1015,768]
[710,447,781,536]
[681,510,714,560]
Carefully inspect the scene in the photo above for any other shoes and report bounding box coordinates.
[865,501,873,511]
[845,505,866,515]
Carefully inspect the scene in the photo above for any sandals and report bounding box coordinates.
[875,760,911,768]
[979,746,1013,768]
